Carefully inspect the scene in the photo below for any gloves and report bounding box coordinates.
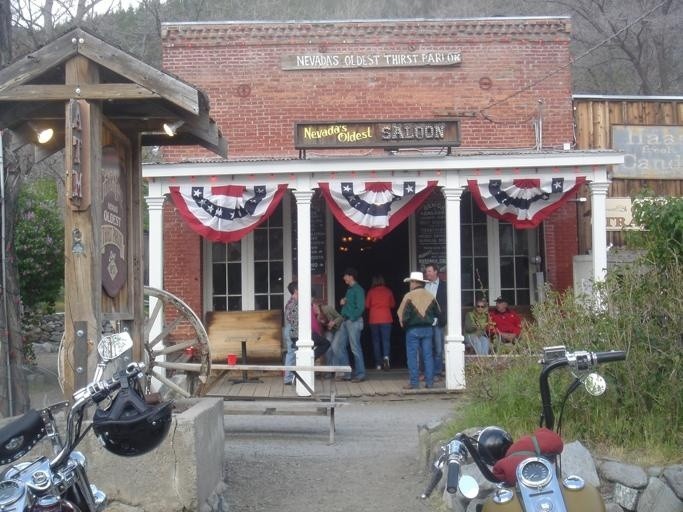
[432,317,438,326]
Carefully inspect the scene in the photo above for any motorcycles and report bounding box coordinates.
[422,351,627,512]
[1,331,146,512]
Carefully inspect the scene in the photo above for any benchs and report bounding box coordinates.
[183,361,352,446]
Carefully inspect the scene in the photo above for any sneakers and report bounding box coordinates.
[316,373,364,382]
[376,356,390,371]
[286,380,295,385]
[404,374,443,390]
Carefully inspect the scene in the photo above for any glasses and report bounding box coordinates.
[477,305,484,308]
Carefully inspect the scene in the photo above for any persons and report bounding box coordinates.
[486,296,522,345]
[418,263,447,381]
[365,272,396,370]
[282,282,344,385]
[330,268,367,383]
[463,299,491,354]
[397,271,441,389]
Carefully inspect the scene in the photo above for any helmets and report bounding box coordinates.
[94,400,173,456]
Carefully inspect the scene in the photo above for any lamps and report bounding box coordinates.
[164,118,184,136]
[26,121,53,144]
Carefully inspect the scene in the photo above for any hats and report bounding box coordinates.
[494,297,510,303]
[403,272,430,283]
